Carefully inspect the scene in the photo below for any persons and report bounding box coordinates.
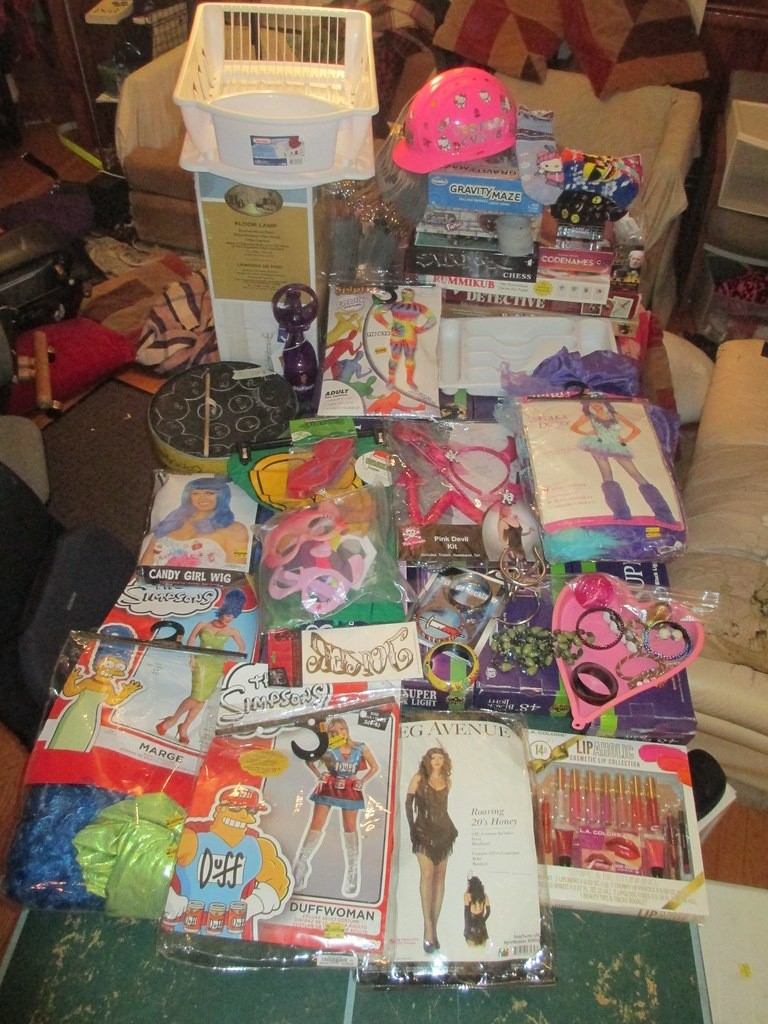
[497,505,535,562]
[462,874,493,944]
[291,719,378,897]
[405,748,458,951]
[572,398,678,525]
[155,588,245,745]
[141,477,248,565]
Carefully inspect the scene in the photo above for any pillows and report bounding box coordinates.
[663,331,715,425]
[7,316,137,417]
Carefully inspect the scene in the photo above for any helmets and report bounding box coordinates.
[392,67,516,174]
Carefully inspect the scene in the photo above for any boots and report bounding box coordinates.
[343,830,359,893]
[292,829,322,889]
[638,484,675,524]
[602,481,632,520]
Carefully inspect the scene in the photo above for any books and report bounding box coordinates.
[407,214,642,319]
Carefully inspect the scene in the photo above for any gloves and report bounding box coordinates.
[447,813,458,839]
[405,793,418,844]
[483,905,490,920]
[463,905,470,936]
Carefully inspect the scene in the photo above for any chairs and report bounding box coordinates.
[489,67,701,319]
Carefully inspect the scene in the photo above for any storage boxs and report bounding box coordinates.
[170,1,383,174]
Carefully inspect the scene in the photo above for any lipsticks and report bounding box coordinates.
[585,769,597,824]
[543,797,553,857]
[602,773,612,824]
[555,829,573,867]
[557,767,566,823]
[631,775,644,828]
[615,772,628,827]
[645,833,665,878]
[647,776,660,827]
[569,767,580,825]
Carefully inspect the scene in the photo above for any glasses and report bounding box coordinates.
[265,507,376,612]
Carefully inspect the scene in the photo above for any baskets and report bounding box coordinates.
[174,4,379,173]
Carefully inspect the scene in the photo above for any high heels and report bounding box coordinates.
[175,724,190,744]
[156,716,172,735]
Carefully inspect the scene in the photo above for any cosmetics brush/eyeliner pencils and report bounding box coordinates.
[678,806,690,873]
[667,808,679,878]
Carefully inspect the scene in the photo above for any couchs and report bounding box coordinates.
[114,0,447,254]
[658,330,768,787]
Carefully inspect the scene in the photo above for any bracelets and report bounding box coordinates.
[415,546,545,691]
[570,603,694,703]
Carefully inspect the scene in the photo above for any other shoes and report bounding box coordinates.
[424,939,441,953]
